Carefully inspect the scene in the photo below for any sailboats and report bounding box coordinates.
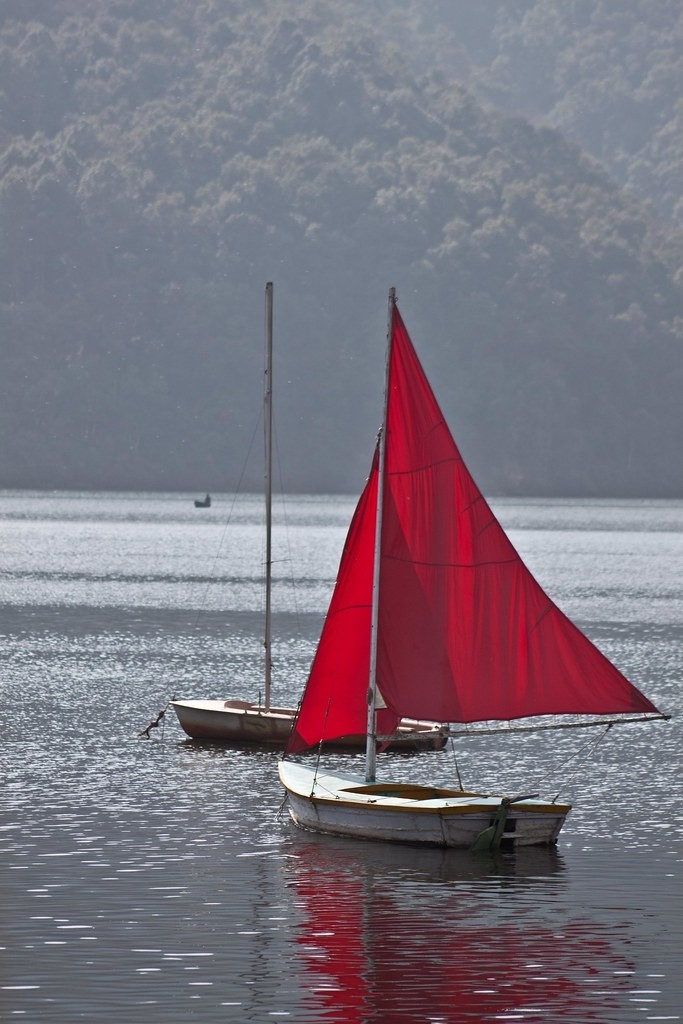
[167,280,452,752]
[276,282,675,854]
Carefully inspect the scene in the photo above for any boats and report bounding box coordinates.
[195,493,212,508]
